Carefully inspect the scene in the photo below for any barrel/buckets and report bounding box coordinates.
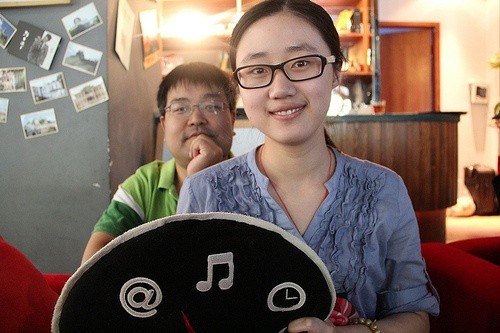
[464,165,497,215]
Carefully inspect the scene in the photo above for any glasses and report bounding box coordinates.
[164,101,230,117]
[232,54,336,89]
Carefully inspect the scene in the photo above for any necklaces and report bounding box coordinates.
[259,142,333,236]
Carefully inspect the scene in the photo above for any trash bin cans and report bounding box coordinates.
[465,164,495,215]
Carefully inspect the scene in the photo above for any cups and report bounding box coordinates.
[371,100,386,116]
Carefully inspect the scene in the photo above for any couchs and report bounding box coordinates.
[0,232,500,333]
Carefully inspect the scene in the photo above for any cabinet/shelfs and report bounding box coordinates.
[160,0,380,116]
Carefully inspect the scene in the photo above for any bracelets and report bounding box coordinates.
[351,318,382,333]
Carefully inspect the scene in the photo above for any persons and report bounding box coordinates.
[27,34,52,67]
[80,61,238,265]
[176,0,440,333]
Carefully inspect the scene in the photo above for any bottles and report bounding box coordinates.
[339,8,353,29]
[352,9,361,32]
